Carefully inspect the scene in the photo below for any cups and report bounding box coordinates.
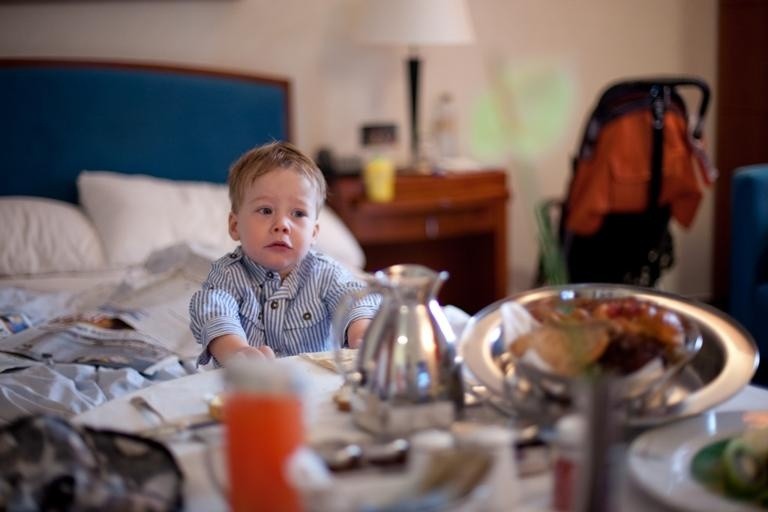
[221,362,304,512]
[363,158,396,203]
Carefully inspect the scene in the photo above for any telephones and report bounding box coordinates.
[317,149,362,178]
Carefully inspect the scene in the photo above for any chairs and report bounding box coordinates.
[731,165,767,358]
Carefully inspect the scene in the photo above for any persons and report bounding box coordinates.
[188,140,380,368]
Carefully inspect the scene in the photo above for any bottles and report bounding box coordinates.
[332,264,464,438]
[546,415,594,511]
[434,94,458,173]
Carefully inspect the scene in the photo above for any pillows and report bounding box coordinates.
[75,170,366,270]
[0,195,104,278]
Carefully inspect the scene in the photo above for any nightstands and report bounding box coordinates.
[325,166,511,314]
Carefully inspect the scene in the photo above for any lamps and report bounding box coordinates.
[355,0,477,173]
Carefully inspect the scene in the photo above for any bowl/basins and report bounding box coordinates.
[509,297,703,414]
[458,280,759,430]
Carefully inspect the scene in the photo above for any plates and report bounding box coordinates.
[169,427,334,511]
[626,410,766,512]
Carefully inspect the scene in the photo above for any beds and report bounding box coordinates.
[1,52,445,430]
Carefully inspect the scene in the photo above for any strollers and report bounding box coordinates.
[526,71,715,289]
